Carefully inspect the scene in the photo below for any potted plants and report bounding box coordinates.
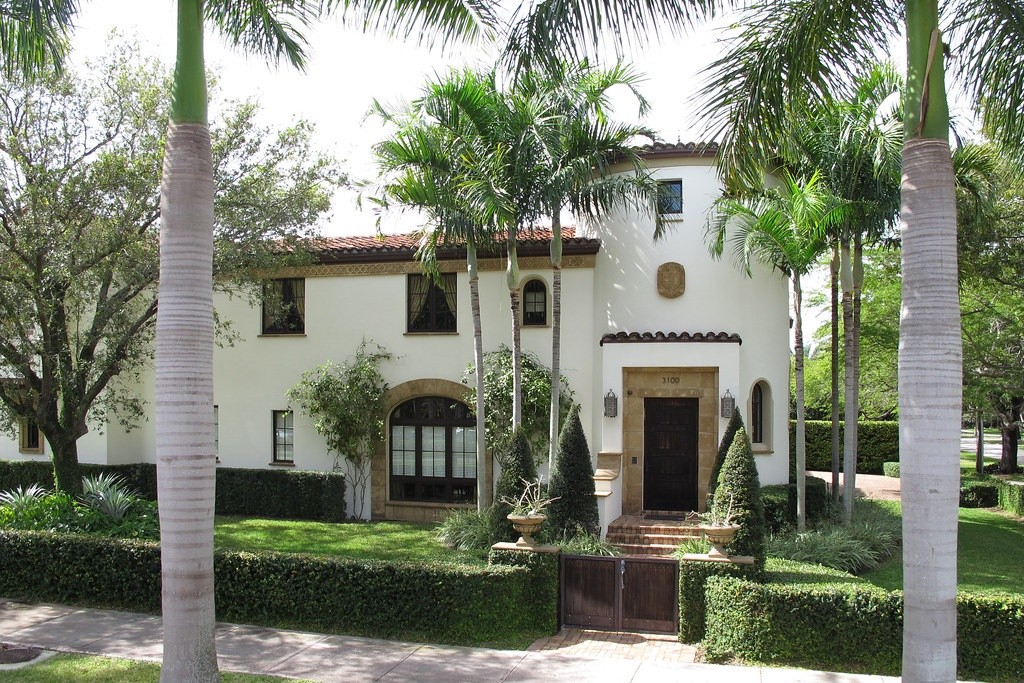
[500,474,563,545]
[686,492,744,558]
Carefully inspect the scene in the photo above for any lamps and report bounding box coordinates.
[722,389,736,419]
[604,388,618,417]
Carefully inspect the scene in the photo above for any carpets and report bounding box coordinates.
[645,515,685,521]
